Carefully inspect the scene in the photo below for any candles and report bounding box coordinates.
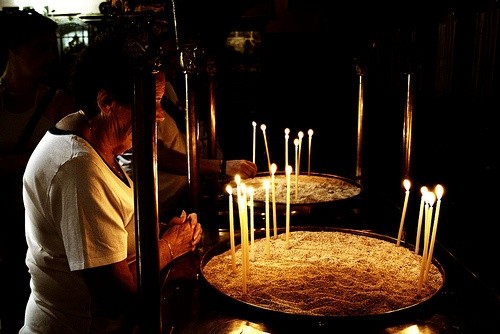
[261,124,270,169]
[307,129,314,175]
[252,121,256,164]
[415,184,443,296]
[225,163,277,294]
[396,179,411,247]
[286,165,292,250]
[285,128,290,165]
[294,131,304,200]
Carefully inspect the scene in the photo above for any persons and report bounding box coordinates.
[0,16,78,334]
[117,41,257,233]
[18,37,202,334]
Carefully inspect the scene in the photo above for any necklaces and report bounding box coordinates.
[85,119,124,179]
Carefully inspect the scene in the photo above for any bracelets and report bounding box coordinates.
[219,160,227,181]
[161,238,175,262]
